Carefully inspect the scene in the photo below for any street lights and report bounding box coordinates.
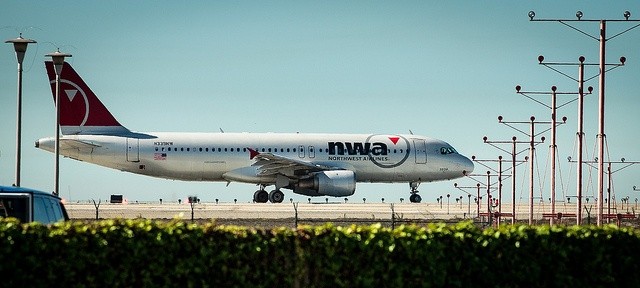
[528,11,638,230]
[473,155,530,228]
[498,115,568,228]
[516,85,594,228]
[464,171,511,232]
[538,54,626,227]
[3,32,37,188]
[484,136,554,229]
[44,47,73,196]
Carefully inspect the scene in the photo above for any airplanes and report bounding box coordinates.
[34,61,475,204]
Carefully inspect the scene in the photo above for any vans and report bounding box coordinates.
[1,186,68,228]
